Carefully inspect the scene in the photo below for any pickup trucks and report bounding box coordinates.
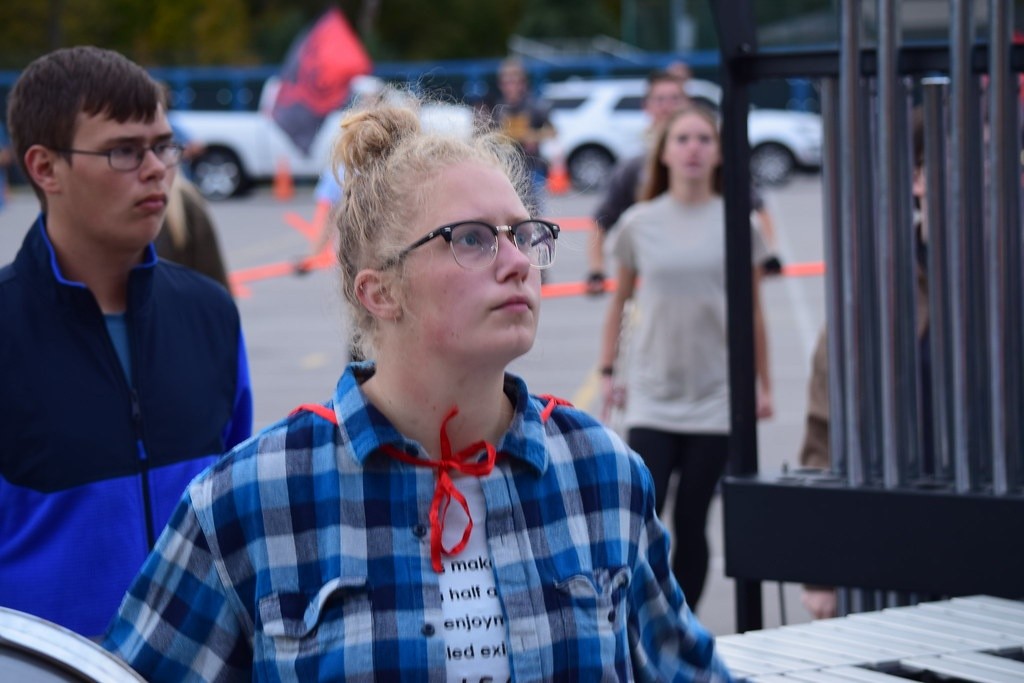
[162,71,475,199]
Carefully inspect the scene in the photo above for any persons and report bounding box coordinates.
[0,45,251,649]
[98,94,752,683]
[295,58,938,635]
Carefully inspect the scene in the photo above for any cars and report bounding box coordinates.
[535,79,825,195]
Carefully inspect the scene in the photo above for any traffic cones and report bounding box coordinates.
[544,151,569,191]
[274,157,293,200]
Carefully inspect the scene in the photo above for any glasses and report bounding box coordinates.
[50,141,185,172]
[377,219,559,270]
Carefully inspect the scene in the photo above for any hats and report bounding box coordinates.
[350,74,385,95]
